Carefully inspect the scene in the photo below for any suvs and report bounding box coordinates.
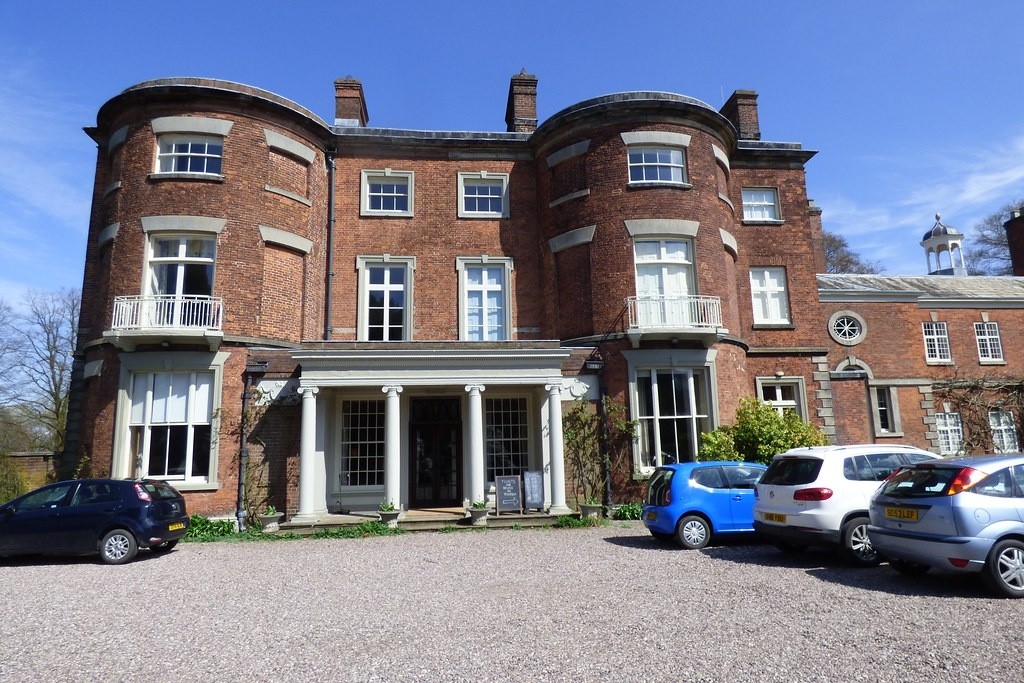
[753,443,948,567]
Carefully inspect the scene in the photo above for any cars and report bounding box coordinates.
[0,478,190,568]
[642,460,775,548]
[869,453,1024,601]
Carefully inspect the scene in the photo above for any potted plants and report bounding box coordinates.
[562,392,640,518]
[256,505,285,533]
[466,501,490,526]
[377,497,400,529]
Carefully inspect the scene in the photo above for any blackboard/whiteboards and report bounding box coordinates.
[523,469,545,514]
[495,475,523,516]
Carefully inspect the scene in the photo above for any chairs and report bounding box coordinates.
[96,485,113,502]
[76,487,97,504]
